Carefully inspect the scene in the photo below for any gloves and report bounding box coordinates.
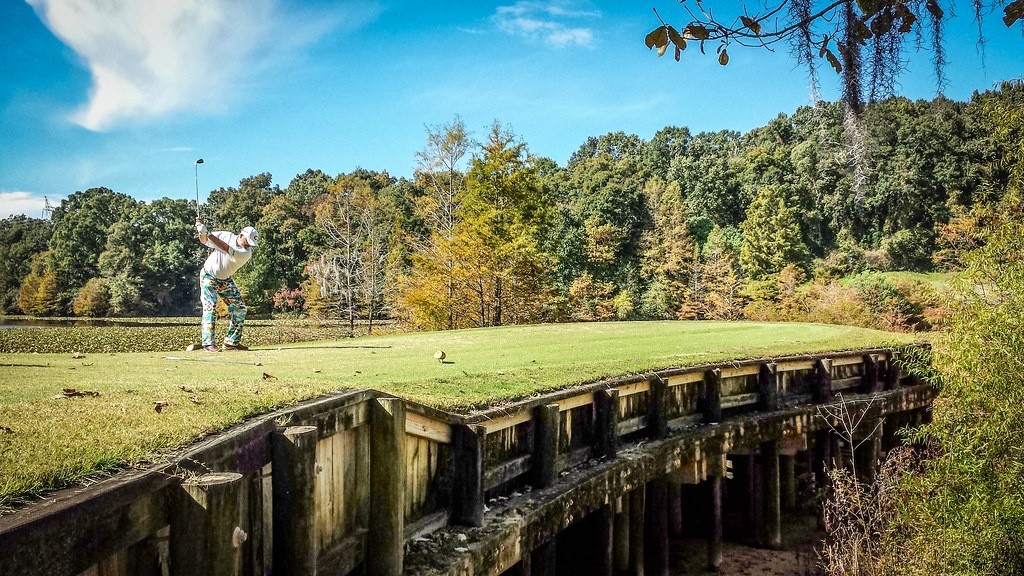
[196,224,212,238]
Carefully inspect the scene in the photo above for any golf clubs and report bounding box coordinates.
[166,356,261,366]
[195,158,204,235]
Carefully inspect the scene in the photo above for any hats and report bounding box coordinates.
[241,226,259,247]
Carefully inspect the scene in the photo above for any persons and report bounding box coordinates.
[195,217,258,352]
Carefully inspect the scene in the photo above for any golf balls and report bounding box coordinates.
[433,349,446,359]
[278,347,282,350]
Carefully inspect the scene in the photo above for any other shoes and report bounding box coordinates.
[204,344,222,352]
[221,342,248,351]
